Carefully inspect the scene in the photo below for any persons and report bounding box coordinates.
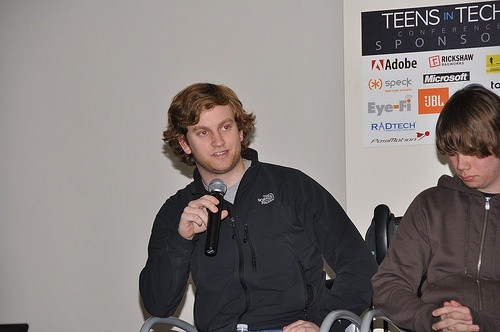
[139,82,377,332]
[370,85,500,332]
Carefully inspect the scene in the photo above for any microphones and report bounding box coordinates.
[205,177,227,257]
